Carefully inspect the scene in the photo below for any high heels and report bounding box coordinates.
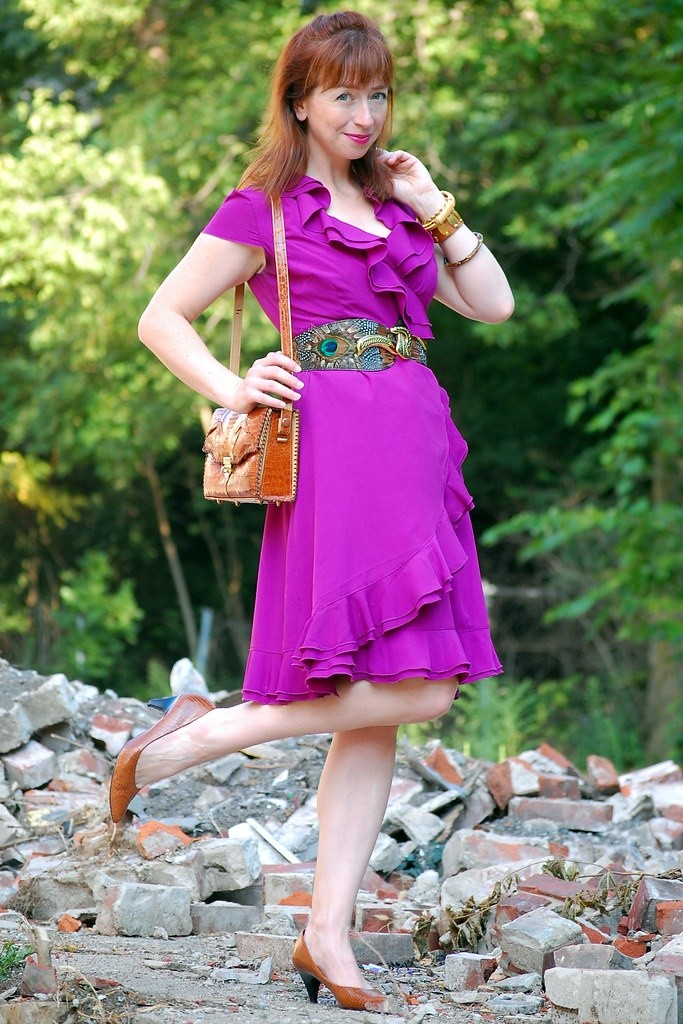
[293,931,385,1010]
[110,695,217,824]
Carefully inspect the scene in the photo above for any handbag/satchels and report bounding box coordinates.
[201,403,300,507]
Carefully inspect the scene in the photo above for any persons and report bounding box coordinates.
[107,12,512,1006]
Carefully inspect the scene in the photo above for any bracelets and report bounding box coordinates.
[414,190,455,230]
[443,232,483,268]
[422,201,463,242]
